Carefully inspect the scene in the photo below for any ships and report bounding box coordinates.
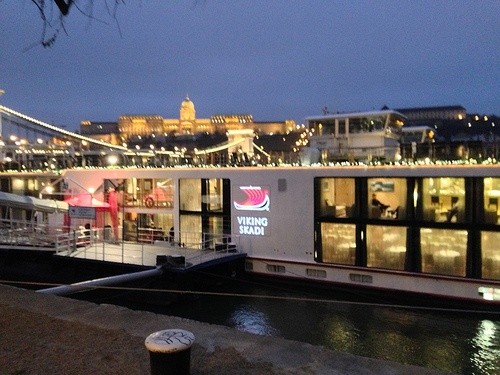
[0,108,499,322]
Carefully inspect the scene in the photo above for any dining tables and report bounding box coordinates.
[320,242,500,279]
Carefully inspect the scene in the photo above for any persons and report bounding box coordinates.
[372,193,390,214]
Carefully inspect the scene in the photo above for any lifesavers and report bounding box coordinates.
[145,197,155,208]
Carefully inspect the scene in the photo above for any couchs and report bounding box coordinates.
[320,199,500,224]
